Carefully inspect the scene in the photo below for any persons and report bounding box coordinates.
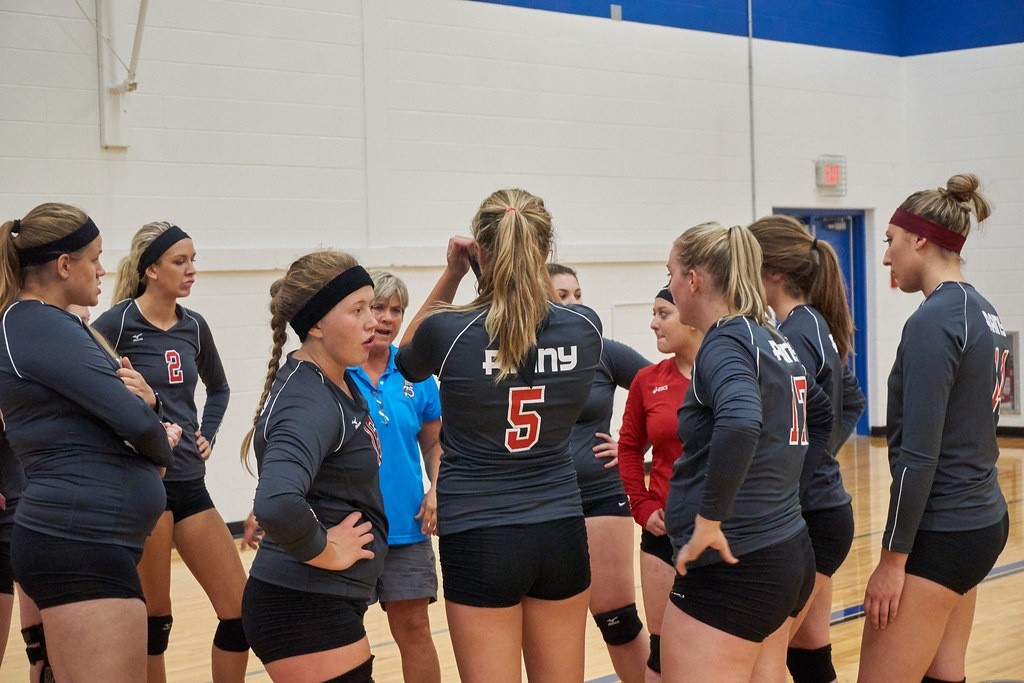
[618,289,705,683]
[856,175,1009,683]
[347,270,440,683]
[93,220,248,683]
[543,264,654,683]
[396,188,604,683]
[748,215,868,683]
[0,204,182,683]
[661,221,837,682]
[242,251,387,683]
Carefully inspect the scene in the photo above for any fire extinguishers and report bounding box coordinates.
[1003,355,1014,403]
